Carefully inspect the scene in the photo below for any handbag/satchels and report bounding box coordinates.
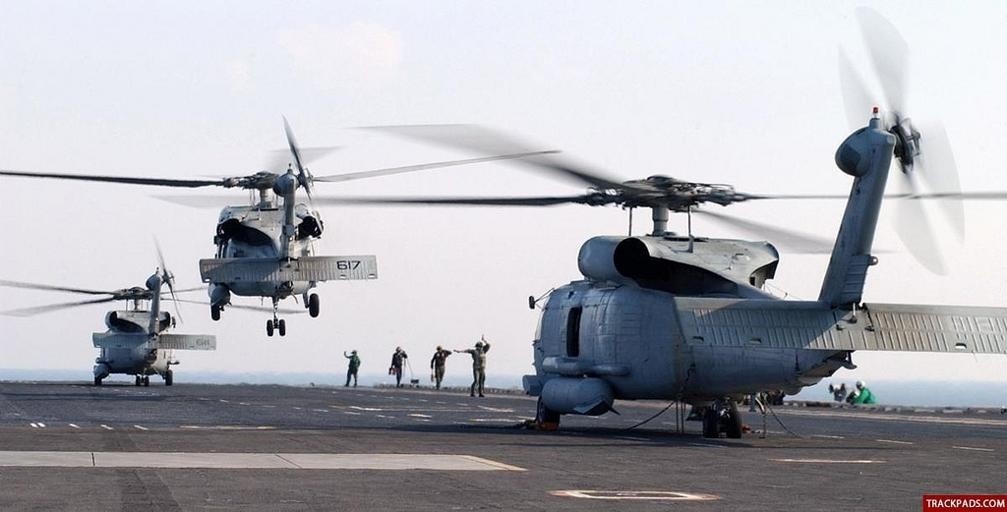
[388,368,395,375]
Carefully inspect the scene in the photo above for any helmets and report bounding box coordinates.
[396,347,401,351]
[436,346,443,350]
[474,341,483,348]
[351,350,357,355]
[855,380,866,389]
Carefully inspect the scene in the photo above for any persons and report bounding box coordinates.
[344,346,361,386]
[829,377,875,410]
[389,346,406,391]
[453,333,491,403]
[430,342,449,392]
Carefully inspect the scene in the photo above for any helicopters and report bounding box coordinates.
[364,9,1006,445]
[0,116,572,336]
[0,237,306,388]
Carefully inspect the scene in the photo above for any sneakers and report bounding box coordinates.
[470,393,484,397]
